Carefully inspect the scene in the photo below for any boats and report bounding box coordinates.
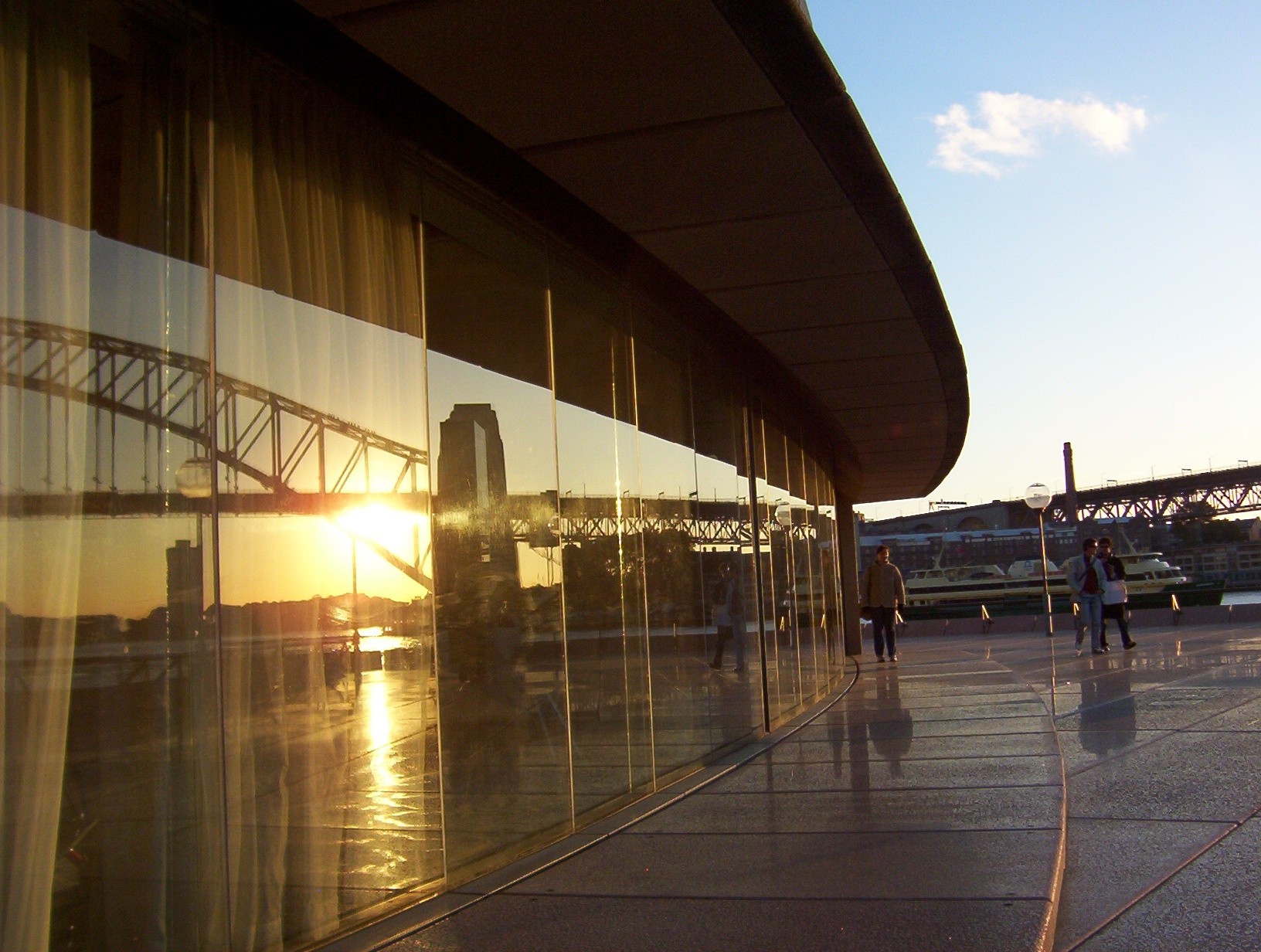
[902,530,1200,619]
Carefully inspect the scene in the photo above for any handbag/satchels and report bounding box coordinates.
[716,579,728,605]
[861,605,872,621]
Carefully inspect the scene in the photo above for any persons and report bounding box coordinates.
[860,544,906,663]
[710,561,751,672]
[810,548,841,664]
[1096,537,1136,652]
[1066,538,1106,653]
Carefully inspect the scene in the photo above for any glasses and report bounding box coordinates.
[1088,545,1099,549]
[1099,545,1109,548]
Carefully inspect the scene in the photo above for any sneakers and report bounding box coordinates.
[889,654,901,660]
[876,655,887,663]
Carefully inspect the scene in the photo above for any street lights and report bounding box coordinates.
[1023,482,1054,636]
[774,502,796,649]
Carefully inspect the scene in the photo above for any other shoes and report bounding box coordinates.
[708,662,722,671]
[1102,646,1111,652]
[732,667,747,673]
[1075,648,1085,657]
[1091,648,1106,654]
[1123,640,1138,650]
